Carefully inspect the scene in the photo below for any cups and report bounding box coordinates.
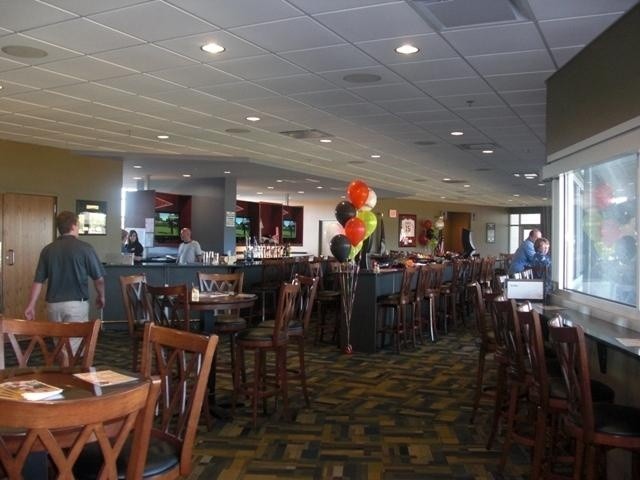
[202,250,220,265]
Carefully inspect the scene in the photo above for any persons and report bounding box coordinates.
[506,229,553,278]
[129,230,142,261]
[121,229,128,254]
[175,227,202,265]
[532,238,552,305]
[23,211,107,371]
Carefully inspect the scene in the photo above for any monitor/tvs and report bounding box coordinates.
[283,218,297,242]
[235,215,255,243]
[154,210,183,243]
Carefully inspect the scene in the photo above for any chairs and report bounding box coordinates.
[467,278,640,479]
[310,239,516,357]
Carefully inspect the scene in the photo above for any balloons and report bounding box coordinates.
[418,220,438,253]
[329,181,379,263]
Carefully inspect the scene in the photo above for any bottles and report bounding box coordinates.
[257,243,291,258]
[227,250,233,265]
[243,236,262,261]
[372,261,380,273]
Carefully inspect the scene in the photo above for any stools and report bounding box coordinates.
[1,375,162,480]
[86,321,220,480]
[141,281,218,434]
[120,272,154,372]
[232,278,301,431]
[1,315,103,368]
[196,269,246,403]
[255,273,319,413]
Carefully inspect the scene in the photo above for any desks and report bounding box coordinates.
[157,290,261,423]
[1,366,138,460]
